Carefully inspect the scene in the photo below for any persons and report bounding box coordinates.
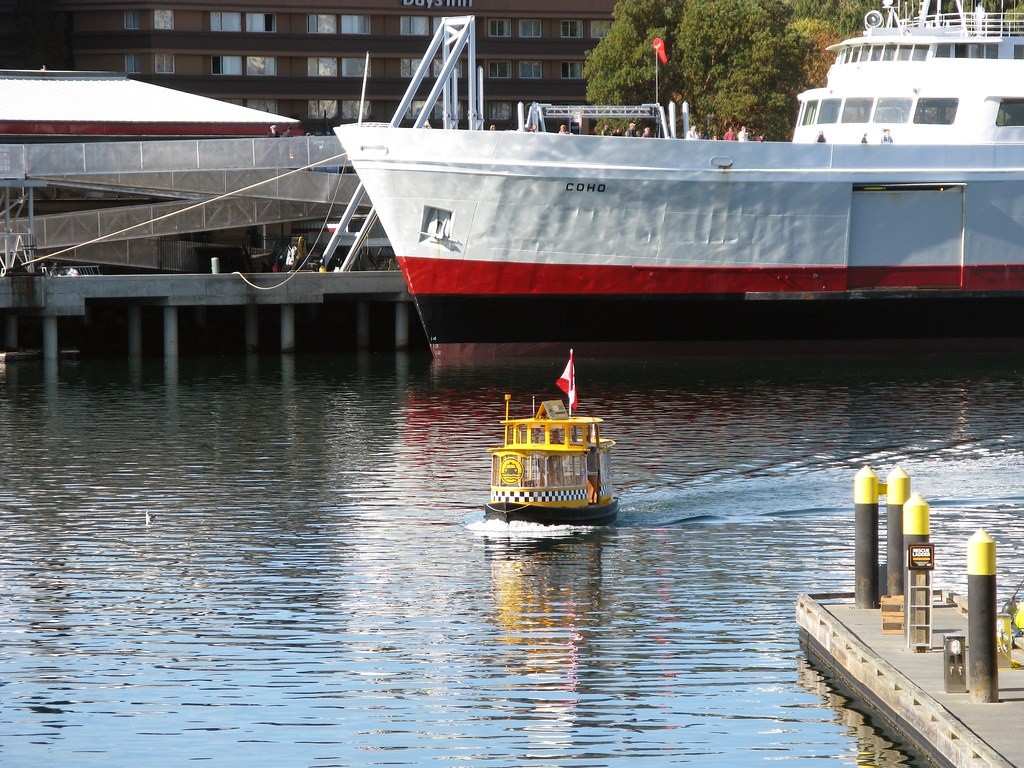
[686,125,765,142]
[37,262,79,277]
[547,430,565,486]
[269,124,293,138]
[490,123,651,137]
[816,129,894,143]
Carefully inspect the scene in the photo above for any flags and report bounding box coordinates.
[555,355,578,410]
[655,38,667,64]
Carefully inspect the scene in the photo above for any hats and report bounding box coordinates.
[628,122,636,128]
[269,125,277,129]
[645,127,651,132]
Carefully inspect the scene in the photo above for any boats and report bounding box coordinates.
[333,1,1023,362]
[483,348,622,530]
